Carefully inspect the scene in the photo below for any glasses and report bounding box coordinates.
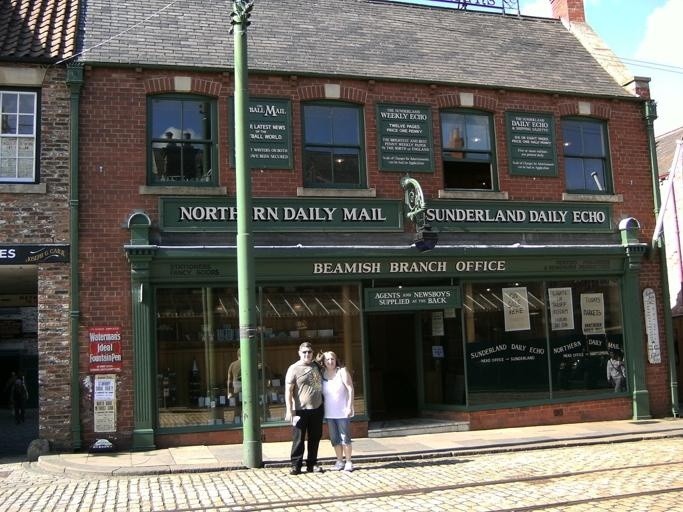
[302,351,311,353]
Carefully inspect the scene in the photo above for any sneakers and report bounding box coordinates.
[306,465,322,472]
[329,462,344,471]
[343,462,353,471]
[288,466,301,475]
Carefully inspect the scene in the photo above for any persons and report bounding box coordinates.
[606,348,627,393]
[226,349,241,423]
[284,342,324,475]
[321,351,355,471]
[5,370,29,425]
